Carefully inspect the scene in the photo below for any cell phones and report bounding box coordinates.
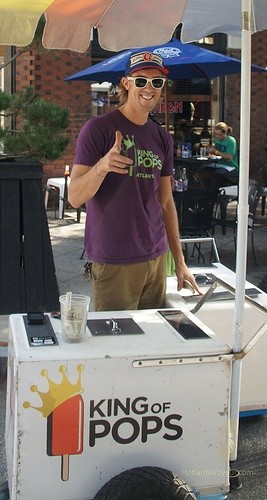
[27,311,44,324]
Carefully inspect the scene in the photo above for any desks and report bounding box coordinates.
[166,263,267,418]
[174,156,221,235]
[5,310,233,500]
[45,178,69,219]
[220,185,267,234]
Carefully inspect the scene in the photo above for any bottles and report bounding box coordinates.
[173,168,188,192]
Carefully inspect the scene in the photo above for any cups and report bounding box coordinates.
[59,294,90,343]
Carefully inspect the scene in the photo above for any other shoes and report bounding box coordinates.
[203,220,215,229]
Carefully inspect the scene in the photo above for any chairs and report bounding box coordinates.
[179,188,220,265]
[209,182,259,264]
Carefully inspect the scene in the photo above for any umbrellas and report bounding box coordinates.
[1,0,267,461]
[63,36,267,82]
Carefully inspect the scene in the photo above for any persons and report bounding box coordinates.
[201,122,238,203]
[67,51,204,311]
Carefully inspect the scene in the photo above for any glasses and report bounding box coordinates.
[127,76,167,90]
[215,133,223,136]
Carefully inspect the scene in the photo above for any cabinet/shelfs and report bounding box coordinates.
[91,95,215,152]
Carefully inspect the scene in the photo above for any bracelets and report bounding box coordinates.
[215,150,219,154]
[94,165,106,177]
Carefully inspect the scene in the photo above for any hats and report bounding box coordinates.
[125,51,169,76]
